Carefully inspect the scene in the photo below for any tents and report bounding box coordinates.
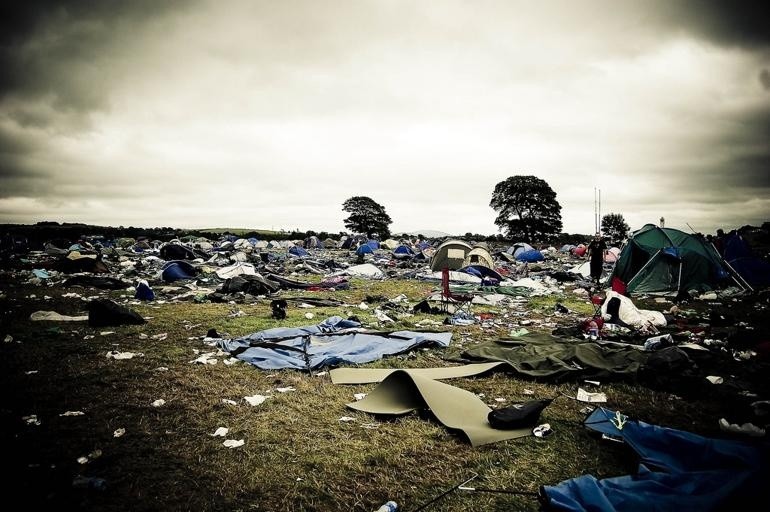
[0,222,769,299]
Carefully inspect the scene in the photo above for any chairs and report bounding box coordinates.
[441,265,475,316]
